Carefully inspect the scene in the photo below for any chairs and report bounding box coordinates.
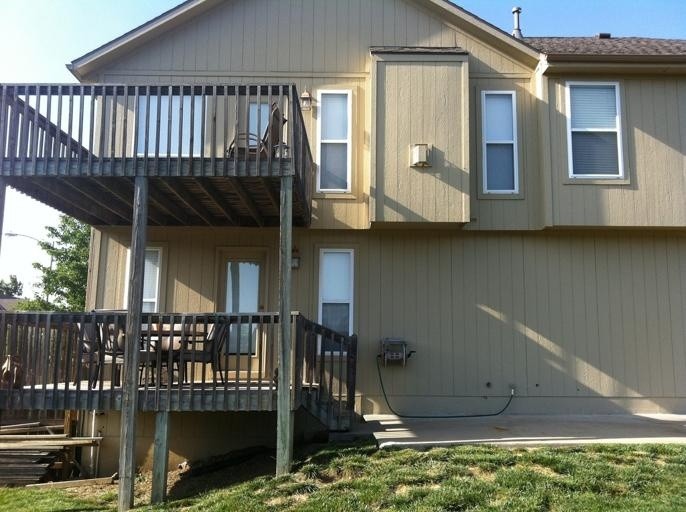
[74,308,230,390]
[226,101,288,160]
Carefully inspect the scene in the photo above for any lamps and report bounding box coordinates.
[291,244,300,269]
[299,83,312,111]
